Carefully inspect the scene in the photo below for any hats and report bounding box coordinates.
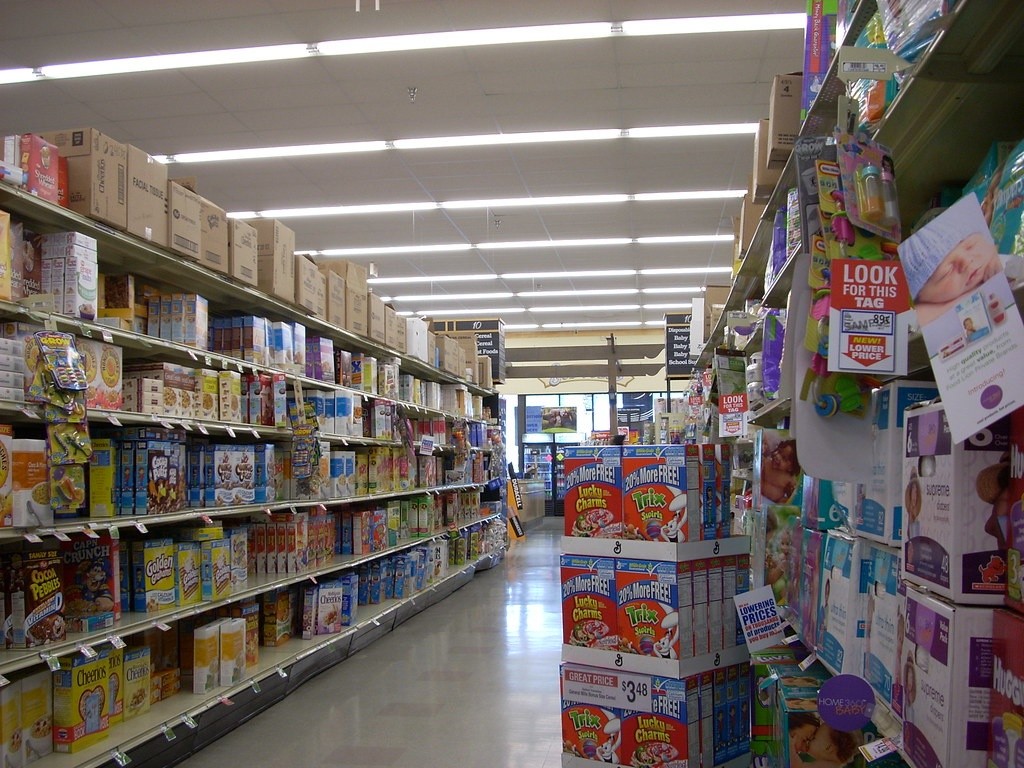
[897,193,988,303]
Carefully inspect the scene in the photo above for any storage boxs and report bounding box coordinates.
[560,0,1024,768]
[2,136,500,767]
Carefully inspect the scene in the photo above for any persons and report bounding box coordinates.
[819,578,830,645]
[760,675,864,768]
[904,466,923,540]
[761,439,801,504]
[896,192,1003,337]
[903,649,917,724]
[896,614,905,685]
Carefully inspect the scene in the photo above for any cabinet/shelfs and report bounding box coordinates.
[657,0,1024,768]
[0,128,508,768]
